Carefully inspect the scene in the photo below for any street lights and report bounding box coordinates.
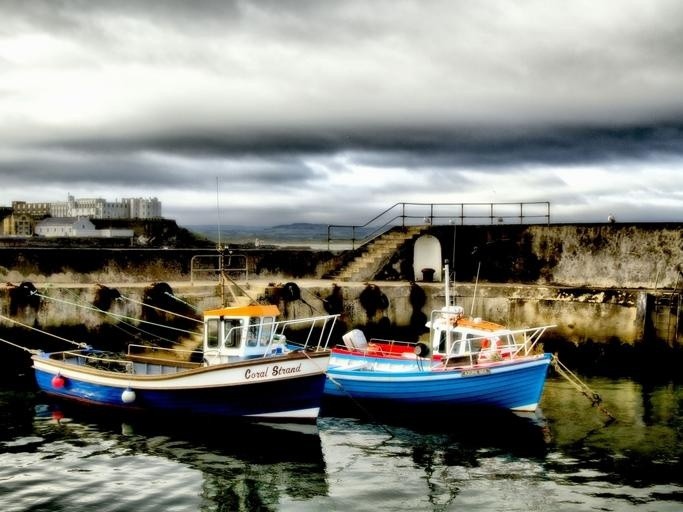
[448,218,460,283]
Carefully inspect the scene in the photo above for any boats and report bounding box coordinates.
[318,408,558,472]
[273,253,560,416]
[29,398,333,501]
[26,174,343,426]
